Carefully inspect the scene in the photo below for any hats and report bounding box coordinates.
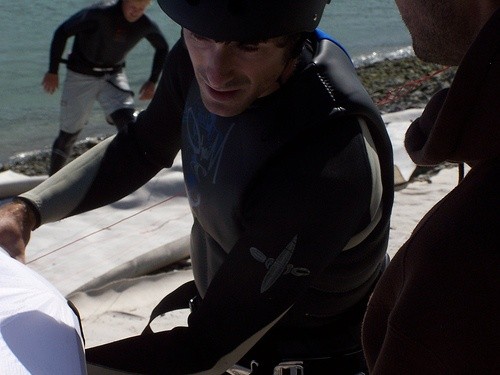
[156,0,328,40]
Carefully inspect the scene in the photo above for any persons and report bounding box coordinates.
[0,0,395,375]
[41,0,169,177]
[361,0,500,375]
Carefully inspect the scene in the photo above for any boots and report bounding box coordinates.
[48,130,81,177]
[110,108,134,134]
